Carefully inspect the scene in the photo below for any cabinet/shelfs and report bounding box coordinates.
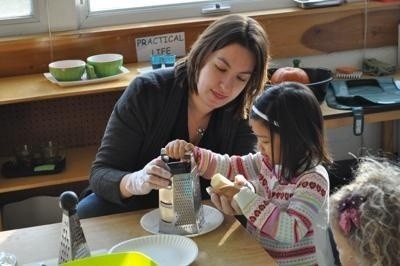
[0,60,178,194]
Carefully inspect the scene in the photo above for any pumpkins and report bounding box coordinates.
[270,68,310,84]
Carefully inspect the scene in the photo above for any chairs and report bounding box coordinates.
[2,195,66,230]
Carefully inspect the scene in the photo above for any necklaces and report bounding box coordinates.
[194,128,206,137]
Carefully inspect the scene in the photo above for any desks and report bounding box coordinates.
[0,198,277,265]
[318,66,400,162]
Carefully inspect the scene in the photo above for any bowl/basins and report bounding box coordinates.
[257,66,335,109]
[86,53,123,75]
[48,58,86,80]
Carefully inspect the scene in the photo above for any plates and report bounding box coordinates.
[137,61,177,74]
[59,251,159,266]
[109,234,198,266]
[140,205,225,237]
[42,65,130,87]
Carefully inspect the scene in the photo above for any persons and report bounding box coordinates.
[75,15,270,219]
[166,81,337,266]
[330,156,400,266]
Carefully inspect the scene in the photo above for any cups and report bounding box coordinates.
[150,54,163,68]
[13,144,32,163]
[163,55,176,67]
[40,141,58,160]
